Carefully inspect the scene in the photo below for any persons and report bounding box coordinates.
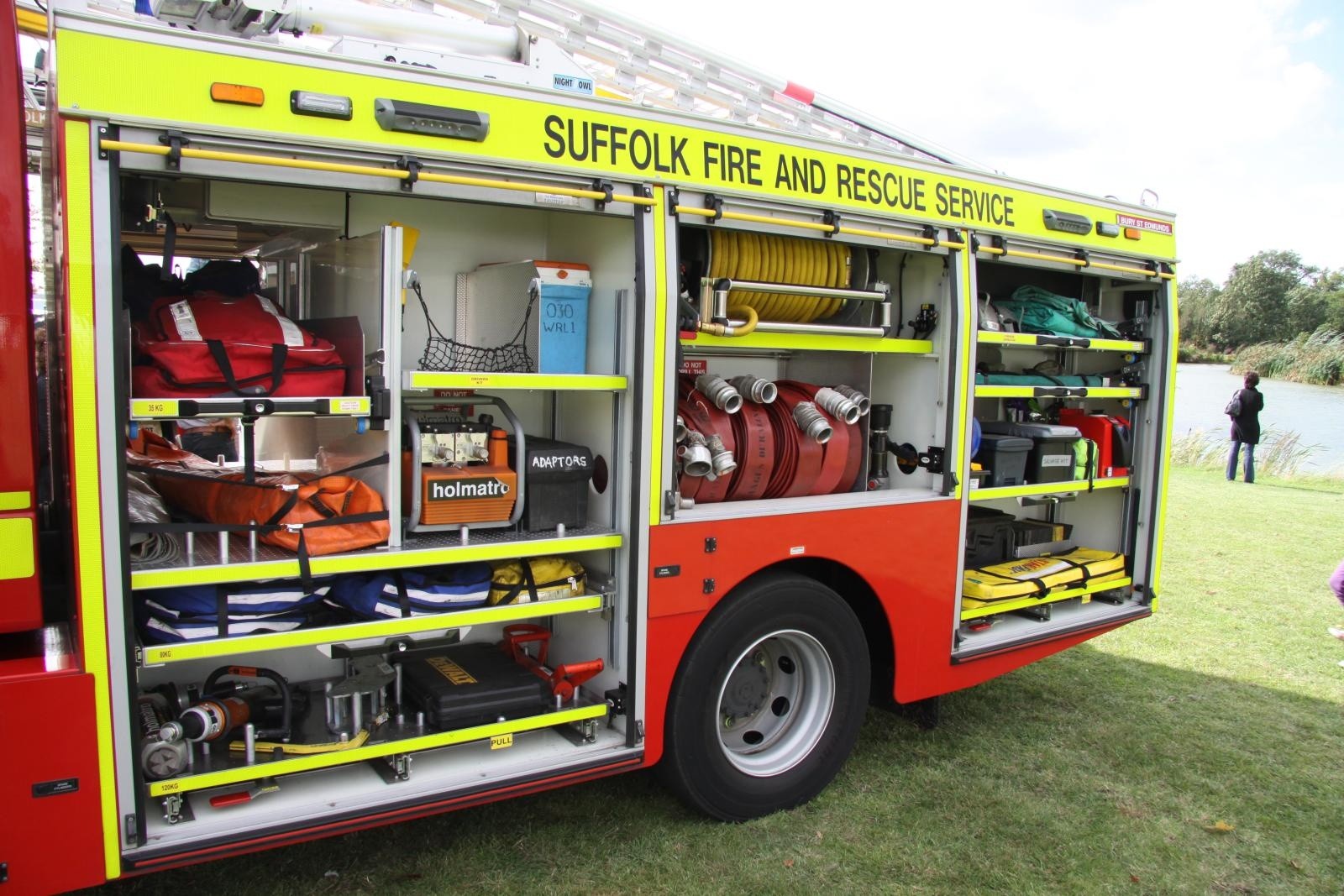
[1224,372,1264,483]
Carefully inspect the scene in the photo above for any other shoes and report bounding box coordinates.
[1328,626,1344,641]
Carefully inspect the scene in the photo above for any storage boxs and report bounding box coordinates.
[970,421,1082,489]
[964,505,1073,570]
[390,643,553,734]
[507,434,594,531]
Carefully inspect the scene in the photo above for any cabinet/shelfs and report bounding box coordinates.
[130,226,628,825]
[960,331,1154,622]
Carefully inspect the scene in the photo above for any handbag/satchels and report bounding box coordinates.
[1225,390,1243,416]
[132,291,344,399]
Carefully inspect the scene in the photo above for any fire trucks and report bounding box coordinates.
[0,2,1180,884]
